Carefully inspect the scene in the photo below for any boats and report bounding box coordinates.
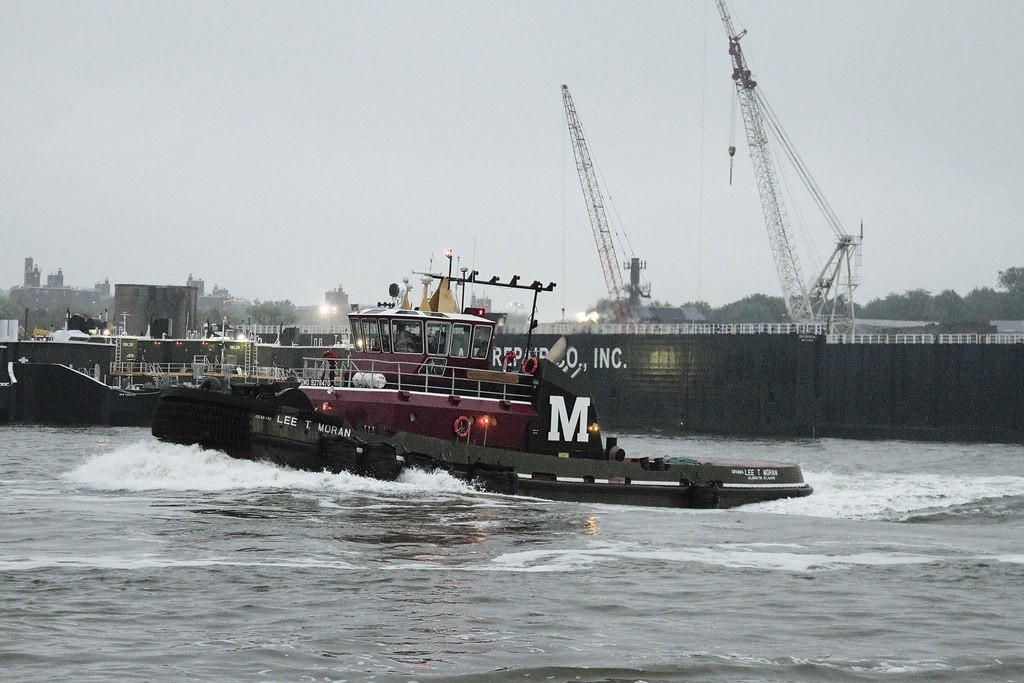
[152,236,814,509]
[6,313,310,430]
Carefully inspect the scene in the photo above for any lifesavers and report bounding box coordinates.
[453,415,471,438]
[519,357,539,375]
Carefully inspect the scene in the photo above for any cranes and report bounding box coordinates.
[713,0,864,338]
[555,82,661,325]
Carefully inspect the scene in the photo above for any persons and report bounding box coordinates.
[432,330,445,349]
